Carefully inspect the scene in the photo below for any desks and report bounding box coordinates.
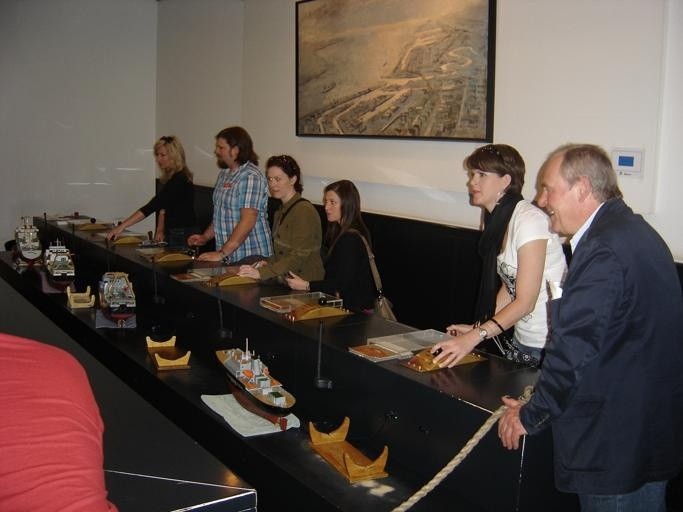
[0,213,541,512]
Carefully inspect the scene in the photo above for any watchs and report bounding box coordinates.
[478,326,489,342]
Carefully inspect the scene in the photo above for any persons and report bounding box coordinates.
[286,179,378,315]
[497,142,682,511]
[107,134,195,240]
[0,330,117,512]
[187,126,268,262]
[237,153,324,289]
[430,144,566,370]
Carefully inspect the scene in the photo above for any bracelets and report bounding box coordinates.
[490,318,505,333]
[219,250,225,257]
[306,282,309,292]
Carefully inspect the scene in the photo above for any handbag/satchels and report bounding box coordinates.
[373,295,397,324]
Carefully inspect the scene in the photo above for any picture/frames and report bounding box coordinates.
[295,1,497,145]
